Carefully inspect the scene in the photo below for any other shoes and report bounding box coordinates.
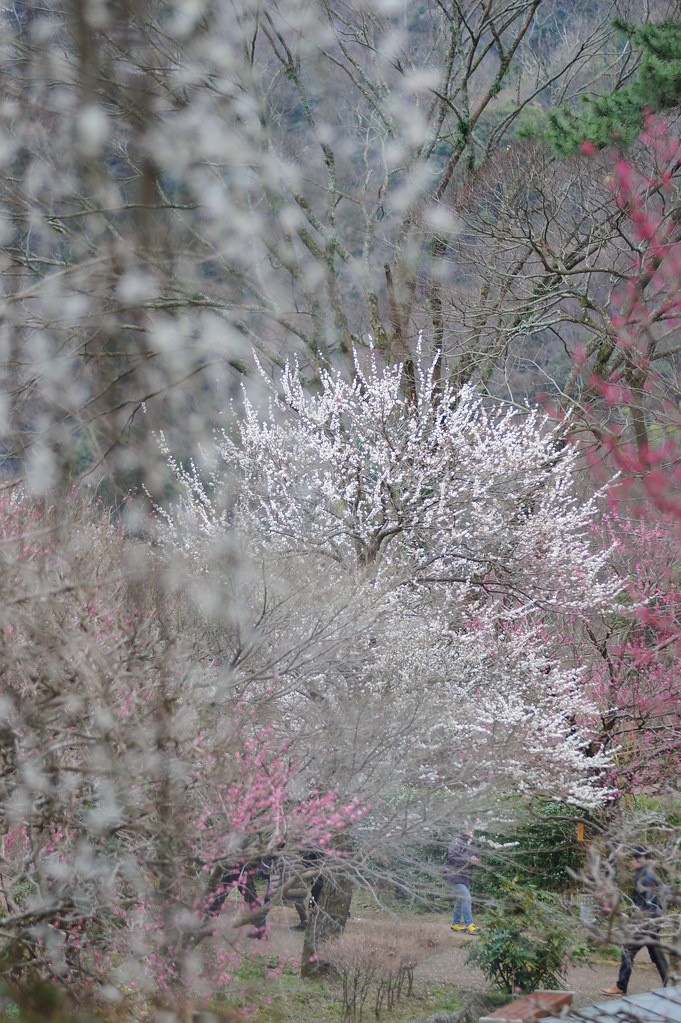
[247,929,265,940]
[290,921,308,931]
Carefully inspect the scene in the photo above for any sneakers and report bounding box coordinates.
[599,983,625,995]
[451,924,466,932]
[466,923,479,934]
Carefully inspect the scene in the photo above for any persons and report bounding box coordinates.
[298,837,322,931]
[260,840,308,930]
[599,845,674,996]
[445,812,482,938]
[196,858,264,939]
